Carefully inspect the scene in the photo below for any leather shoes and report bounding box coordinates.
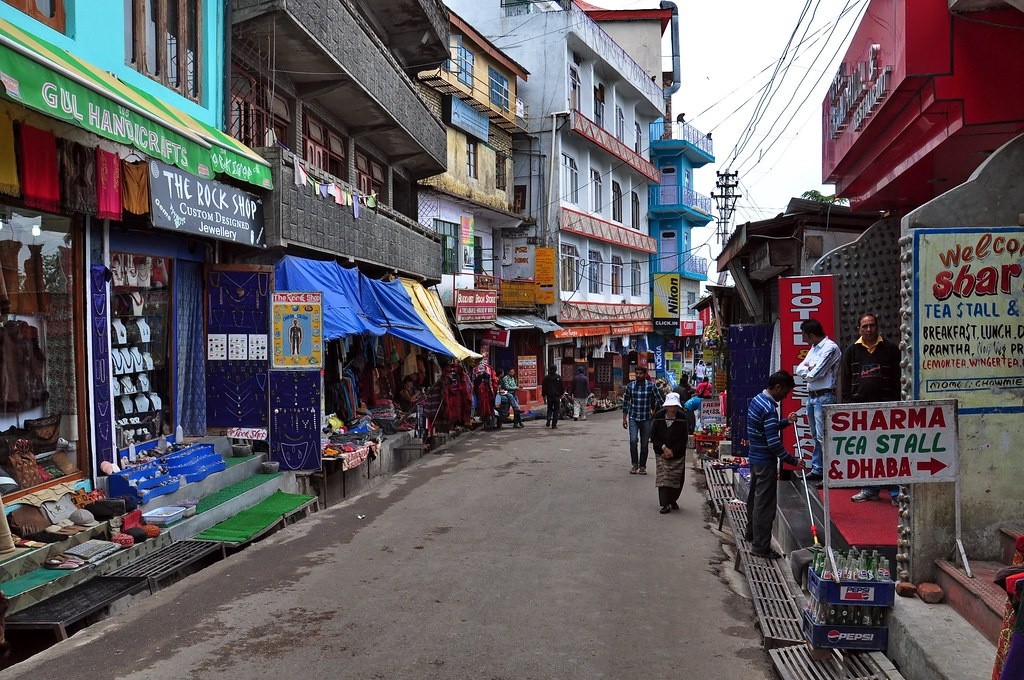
[815,481,823,488]
[797,472,824,481]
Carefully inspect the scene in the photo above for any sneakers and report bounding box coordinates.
[891,496,906,508]
[851,490,879,503]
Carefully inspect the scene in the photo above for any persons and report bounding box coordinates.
[396,376,421,412]
[622,366,664,475]
[440,361,473,424]
[473,363,498,416]
[654,361,712,435]
[651,392,688,514]
[744,370,806,559]
[841,313,902,507]
[495,368,526,429]
[796,319,843,489]
[542,364,565,429]
[569,366,590,421]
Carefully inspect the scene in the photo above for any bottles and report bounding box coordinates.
[807,594,827,633]
[837,606,862,626]
[695,423,707,436]
[708,423,717,436]
[158,435,167,449]
[832,550,857,581]
[855,550,891,582]
[129,443,136,461]
[812,549,833,585]
[717,424,732,437]
[824,603,839,625]
[176,425,183,444]
[862,606,888,626]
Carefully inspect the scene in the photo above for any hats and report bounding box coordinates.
[662,392,683,409]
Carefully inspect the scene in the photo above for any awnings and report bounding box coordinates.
[0,16,274,191]
[552,320,654,338]
[447,314,564,333]
[687,285,734,312]
[275,255,483,362]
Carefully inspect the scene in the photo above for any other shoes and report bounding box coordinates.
[573,417,578,421]
[514,410,525,415]
[552,426,558,428]
[513,422,524,428]
[546,419,551,427]
[638,467,646,475]
[503,416,512,423]
[749,546,780,559]
[630,466,639,474]
[671,501,679,510]
[660,506,671,514]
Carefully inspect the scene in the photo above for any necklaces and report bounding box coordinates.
[270,371,322,473]
[207,272,267,422]
[728,324,773,456]
[91,267,163,470]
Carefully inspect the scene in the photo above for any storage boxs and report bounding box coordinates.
[694,430,725,455]
[802,566,895,652]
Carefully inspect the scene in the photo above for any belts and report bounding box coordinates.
[808,389,834,394]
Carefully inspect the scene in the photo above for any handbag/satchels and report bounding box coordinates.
[698,391,704,398]
